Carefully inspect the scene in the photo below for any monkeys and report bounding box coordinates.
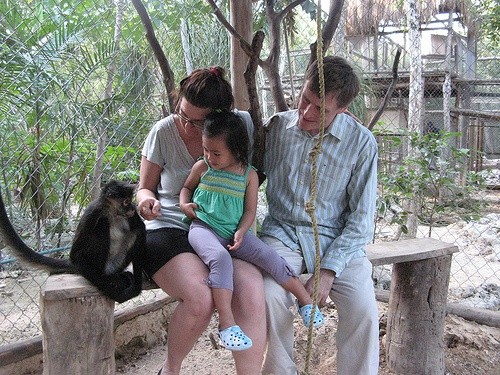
[0,178,148,304]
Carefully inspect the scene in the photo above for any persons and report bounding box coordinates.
[256,55,378,375]
[135,66,363,375]
[420,121,443,170]
[177,106,325,352]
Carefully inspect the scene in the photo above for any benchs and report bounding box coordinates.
[39,237,460,375]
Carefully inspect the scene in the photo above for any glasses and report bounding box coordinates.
[174,97,204,129]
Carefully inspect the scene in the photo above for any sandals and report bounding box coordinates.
[295,299,325,328]
[218,324,252,351]
